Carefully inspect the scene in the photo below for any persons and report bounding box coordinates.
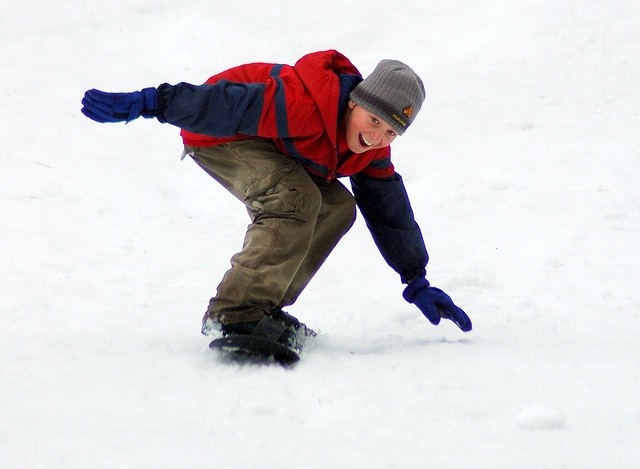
[80,48,472,353]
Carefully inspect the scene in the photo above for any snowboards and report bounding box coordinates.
[209,327,316,368]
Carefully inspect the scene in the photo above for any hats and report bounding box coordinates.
[349,58,426,137]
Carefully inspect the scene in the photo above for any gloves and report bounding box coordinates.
[80,87,159,126]
[403,275,473,334]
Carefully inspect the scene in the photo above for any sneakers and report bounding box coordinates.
[273,305,319,341]
[201,314,302,355]
